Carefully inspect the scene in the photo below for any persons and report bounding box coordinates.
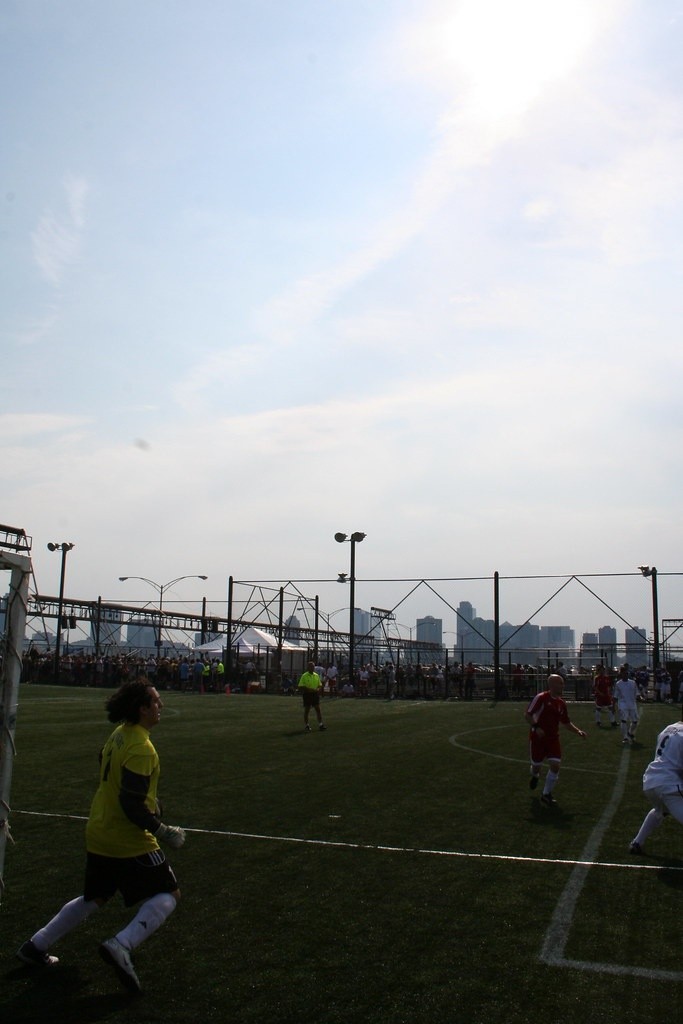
[523,674,586,809]
[20,647,683,704]
[611,669,646,746]
[11,675,188,995]
[628,704,683,855]
[591,666,621,729]
[296,661,328,733]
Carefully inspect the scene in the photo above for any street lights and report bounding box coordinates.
[296,606,362,673]
[118,574,208,659]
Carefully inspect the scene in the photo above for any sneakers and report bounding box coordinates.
[15,936,144,998]
[596,720,637,745]
[529,776,558,805]
[628,840,642,855]
[304,724,328,733]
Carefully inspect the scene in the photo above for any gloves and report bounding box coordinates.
[153,822,187,849]
